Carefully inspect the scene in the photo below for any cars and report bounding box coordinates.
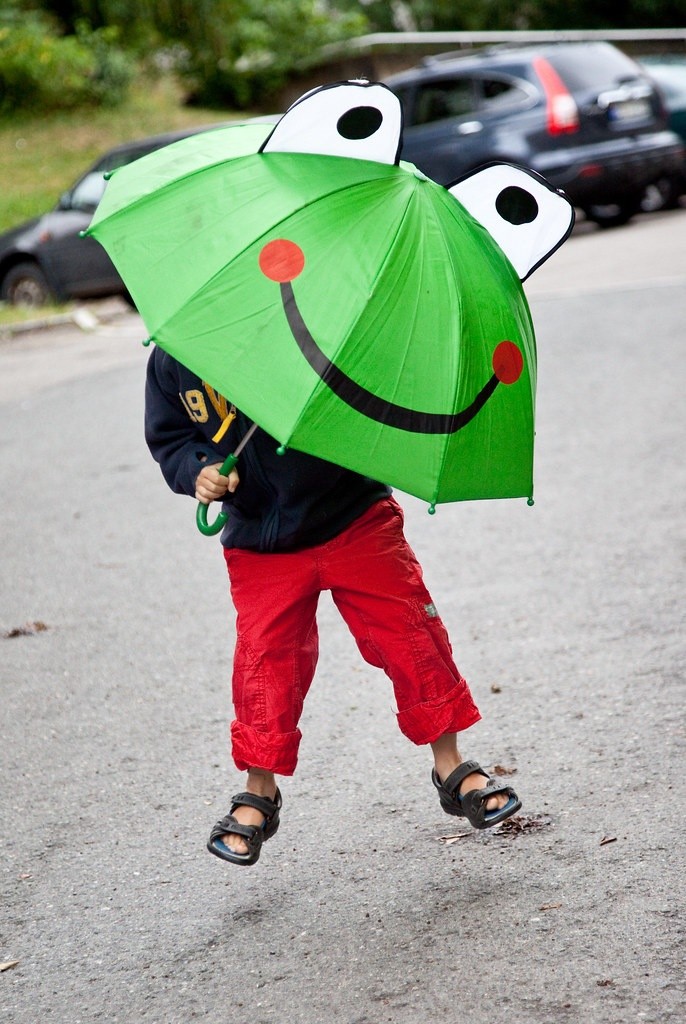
[636,51,683,128]
[1,131,201,313]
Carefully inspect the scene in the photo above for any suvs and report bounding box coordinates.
[373,43,684,228]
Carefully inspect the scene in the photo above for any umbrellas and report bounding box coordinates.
[79,79,576,537]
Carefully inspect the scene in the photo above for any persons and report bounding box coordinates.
[144,345,524,865]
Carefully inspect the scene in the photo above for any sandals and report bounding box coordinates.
[432,760,522,828]
[207,786,283,866]
